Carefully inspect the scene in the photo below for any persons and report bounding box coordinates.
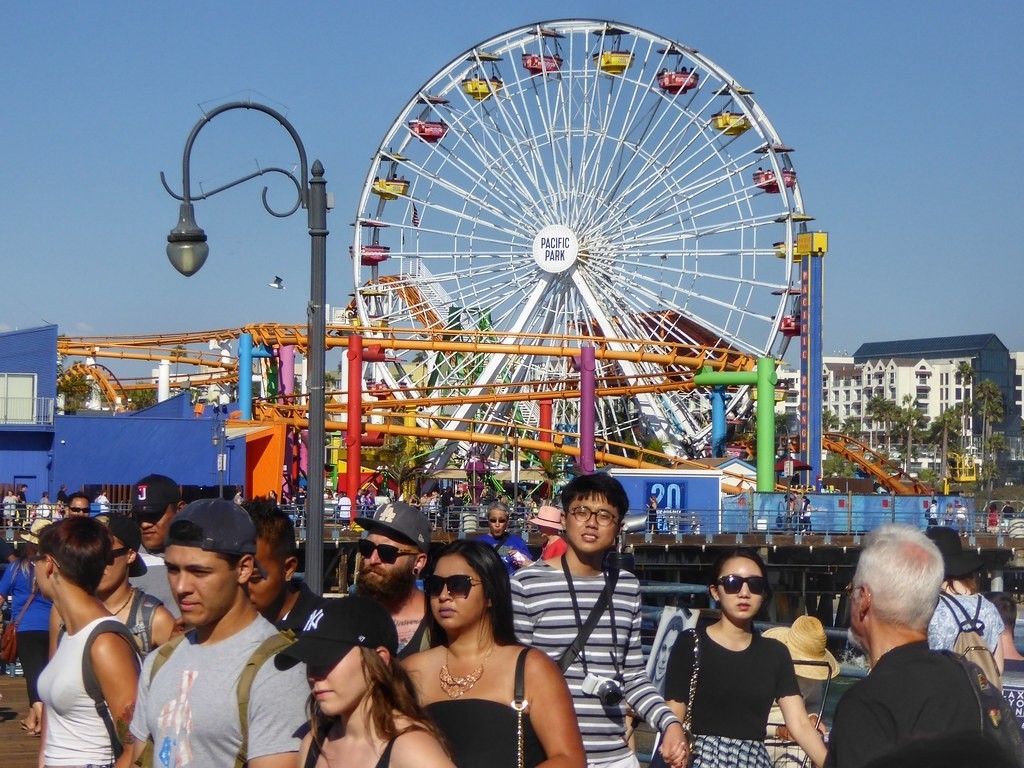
[0,473,1024,768]
[374,66,789,247]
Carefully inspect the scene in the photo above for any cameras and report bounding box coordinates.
[581,673,625,706]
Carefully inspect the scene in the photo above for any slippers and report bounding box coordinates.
[19,721,29,731]
[26,729,41,736]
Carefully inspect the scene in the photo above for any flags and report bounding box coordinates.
[412,203,419,227]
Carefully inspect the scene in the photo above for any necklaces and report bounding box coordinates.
[113,587,133,616]
[439,646,484,698]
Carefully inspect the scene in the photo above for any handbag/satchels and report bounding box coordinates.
[646,732,695,768]
[0,620,18,662]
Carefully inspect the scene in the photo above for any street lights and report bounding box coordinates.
[158,101,332,601]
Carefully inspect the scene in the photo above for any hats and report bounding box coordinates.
[129,473,180,514]
[273,595,398,671]
[20,518,53,544]
[924,526,984,578]
[761,616,840,680]
[165,498,267,580]
[353,501,433,554]
[93,512,148,577]
[530,506,564,530]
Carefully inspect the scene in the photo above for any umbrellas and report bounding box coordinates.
[427,465,467,493]
[493,465,547,481]
[776,455,813,471]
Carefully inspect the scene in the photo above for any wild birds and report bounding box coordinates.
[267,276,286,290]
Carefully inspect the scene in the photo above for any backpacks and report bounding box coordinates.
[925,507,934,520]
[940,590,1004,694]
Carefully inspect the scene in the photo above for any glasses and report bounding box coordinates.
[107,546,129,566]
[706,574,767,595]
[68,506,90,514]
[489,519,506,523]
[423,574,481,599]
[30,555,61,568]
[843,584,871,598]
[358,539,421,564]
[567,505,621,525]
